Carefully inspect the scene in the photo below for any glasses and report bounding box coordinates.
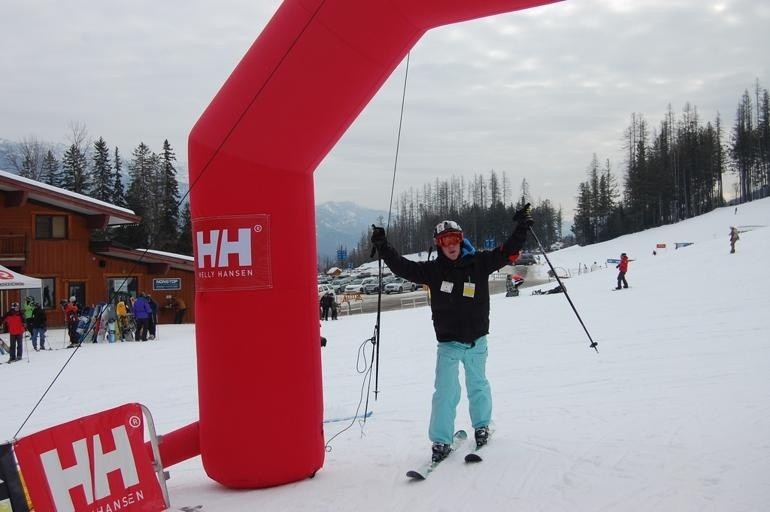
[436,231,464,247]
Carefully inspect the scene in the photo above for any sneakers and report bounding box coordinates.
[474,425,489,446]
[431,442,450,462]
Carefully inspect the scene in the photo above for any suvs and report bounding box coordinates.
[512,253,535,266]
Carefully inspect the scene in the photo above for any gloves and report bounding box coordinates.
[371,227,386,248]
[511,202,535,232]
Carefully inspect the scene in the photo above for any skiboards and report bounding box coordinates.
[406,430,494,479]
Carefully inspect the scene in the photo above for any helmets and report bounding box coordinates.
[433,219,463,240]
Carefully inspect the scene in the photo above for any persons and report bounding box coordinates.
[371,203,534,462]
[616,253,628,289]
[2,291,186,362]
[729,227,739,253]
[506,275,519,297]
[320,292,339,320]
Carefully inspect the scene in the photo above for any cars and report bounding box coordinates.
[317,271,424,300]
